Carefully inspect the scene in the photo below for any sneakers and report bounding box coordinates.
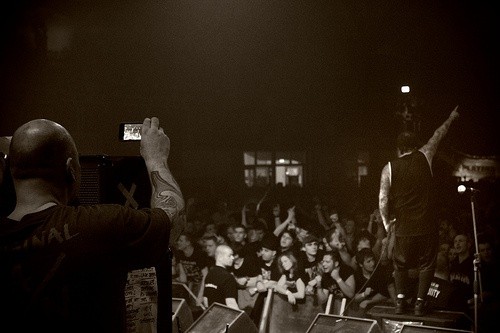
[394,293,410,314]
[414,297,432,316]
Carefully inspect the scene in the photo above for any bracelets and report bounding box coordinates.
[335,278,342,283]
[361,292,365,298]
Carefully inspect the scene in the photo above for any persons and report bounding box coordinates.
[379,103,459,316]
[0,116,184,333]
[171,190,500,333]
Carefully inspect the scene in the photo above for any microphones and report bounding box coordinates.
[458,185,478,193]
[388,218,396,233]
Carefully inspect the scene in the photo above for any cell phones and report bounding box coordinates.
[119,122,143,142]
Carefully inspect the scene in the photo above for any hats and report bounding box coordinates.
[259,233,282,251]
[303,236,321,246]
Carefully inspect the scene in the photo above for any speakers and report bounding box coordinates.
[305,313,381,333]
[185,302,259,333]
[171,298,193,333]
